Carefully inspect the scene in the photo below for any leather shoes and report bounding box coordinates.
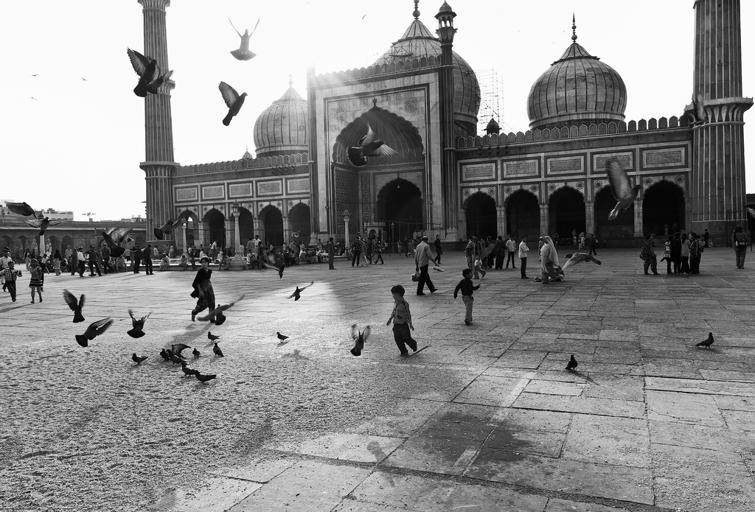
[417,289,437,295]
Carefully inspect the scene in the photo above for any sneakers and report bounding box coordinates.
[475,272,485,279]
[401,343,417,356]
[192,310,195,322]
[31,299,42,303]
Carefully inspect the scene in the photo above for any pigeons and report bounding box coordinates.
[558,354,580,371]
[563,247,607,283]
[345,123,405,174]
[346,322,372,355]
[127,343,230,385]
[284,280,316,303]
[50,287,152,349]
[196,294,248,327]
[206,330,219,343]
[98,214,178,258]
[264,260,288,278]
[127,18,260,128]
[24,212,62,236]
[276,331,292,344]
[601,155,646,224]
[691,330,716,354]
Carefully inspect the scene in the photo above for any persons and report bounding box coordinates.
[464,234,517,280]
[453,268,480,325]
[386,285,417,357]
[643,233,660,275]
[413,234,442,296]
[190,257,216,323]
[239,235,385,270]
[733,226,747,269]
[534,236,558,284]
[570,231,597,256]
[0,239,229,304]
[662,229,710,275]
[518,234,530,279]
